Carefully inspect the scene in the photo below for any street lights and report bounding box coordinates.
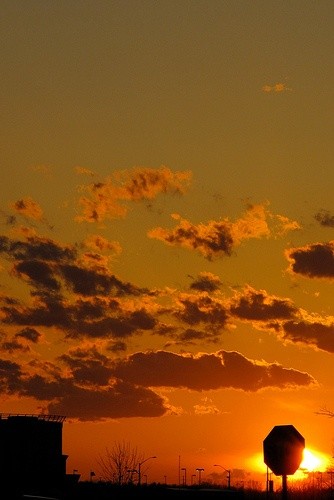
[196,468,205,484]
[213,464,231,487]
[181,468,186,484]
[113,472,116,482]
[138,456,157,483]
[165,475,167,484]
